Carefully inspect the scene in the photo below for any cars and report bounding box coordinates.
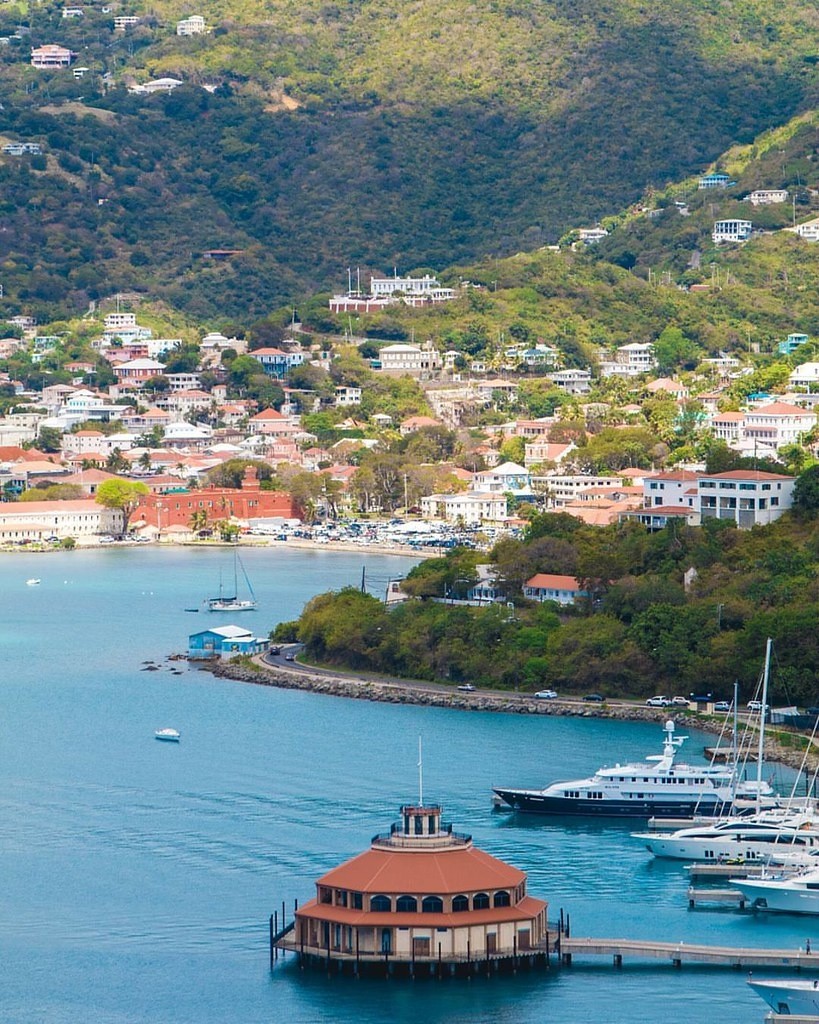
[582,692,607,703]
[805,706,819,715]
[98,534,151,543]
[691,694,713,703]
[670,696,691,707]
[457,683,475,691]
[713,700,730,711]
[534,690,557,700]
[646,695,672,708]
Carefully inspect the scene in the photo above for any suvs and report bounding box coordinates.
[746,700,770,711]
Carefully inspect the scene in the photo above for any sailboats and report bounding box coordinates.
[206,541,259,611]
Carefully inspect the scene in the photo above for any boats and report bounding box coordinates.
[629,633,819,914]
[746,979,819,1018]
[490,720,819,821]
[154,727,181,742]
